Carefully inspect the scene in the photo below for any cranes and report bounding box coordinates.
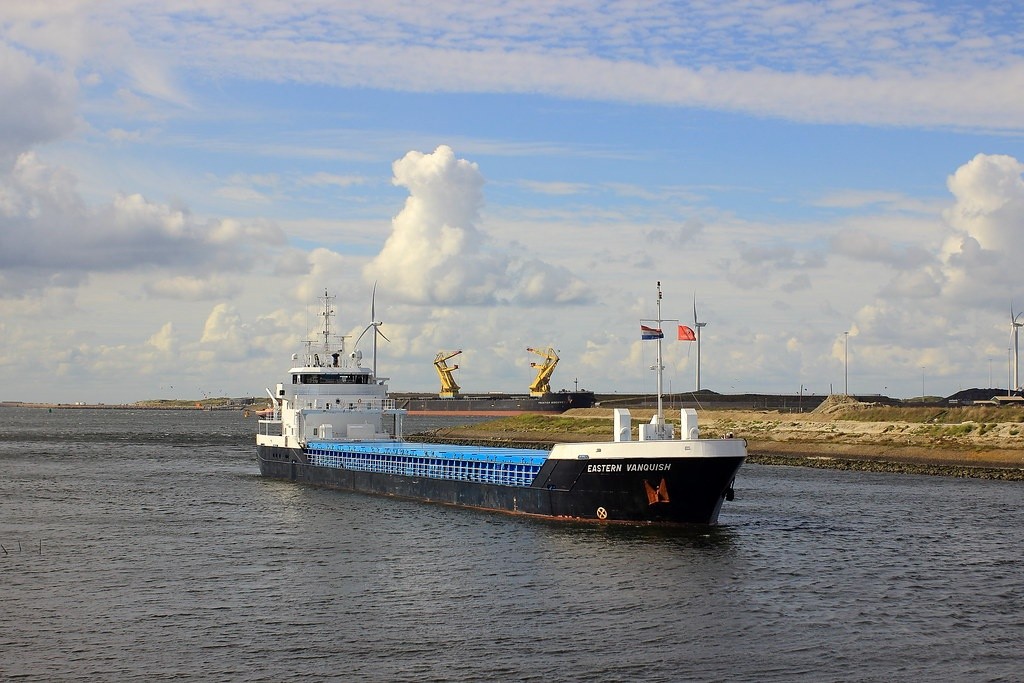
[527,346,561,399]
[432,350,464,399]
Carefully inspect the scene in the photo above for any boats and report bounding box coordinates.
[393,391,598,417]
[251,285,752,532]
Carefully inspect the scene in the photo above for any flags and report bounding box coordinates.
[677,325,696,341]
[641,325,664,340]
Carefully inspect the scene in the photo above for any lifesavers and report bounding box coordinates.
[358,399,361,403]
[726,488,735,502]
